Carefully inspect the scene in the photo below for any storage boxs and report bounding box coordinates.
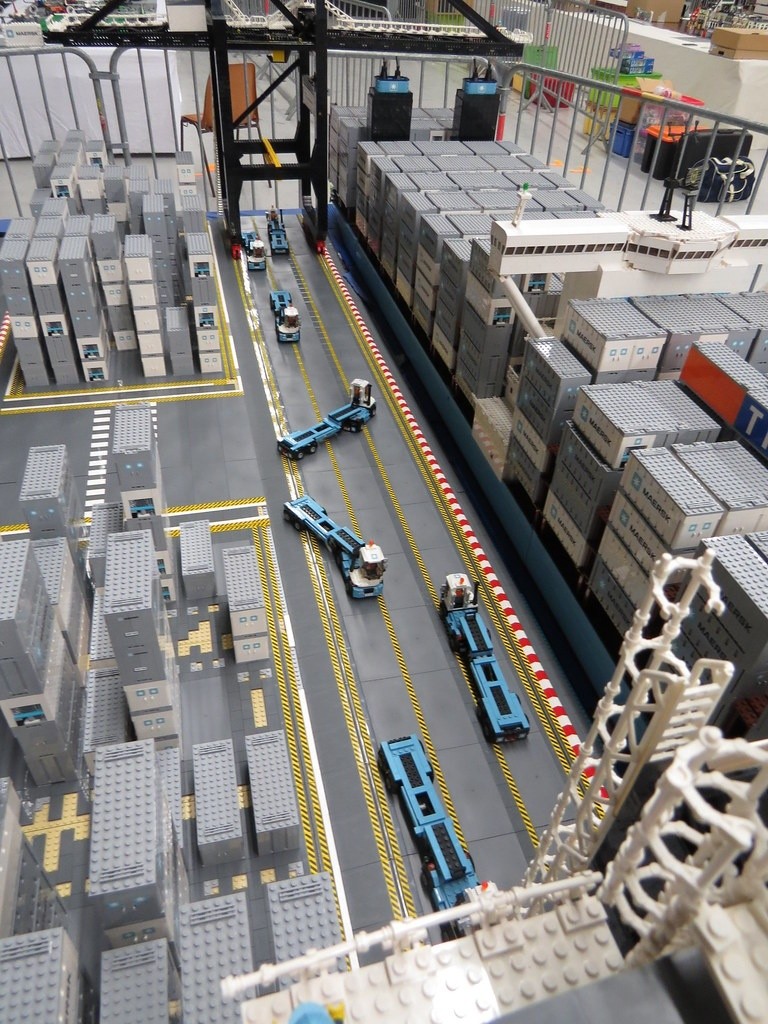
[626,0,686,23]
[640,123,711,179]
[529,72,575,111]
[710,44,768,60]
[608,120,637,158]
[591,68,661,108]
[584,100,619,143]
[712,26,768,51]
[616,75,678,123]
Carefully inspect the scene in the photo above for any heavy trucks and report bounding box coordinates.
[284,495,388,600]
[265,204,289,255]
[277,378,377,460]
[241,230,266,272]
[378,733,499,940]
[438,572,531,743]
[269,290,301,343]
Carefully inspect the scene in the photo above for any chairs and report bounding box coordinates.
[180,63,272,196]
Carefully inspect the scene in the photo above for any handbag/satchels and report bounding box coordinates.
[686,156,755,203]
